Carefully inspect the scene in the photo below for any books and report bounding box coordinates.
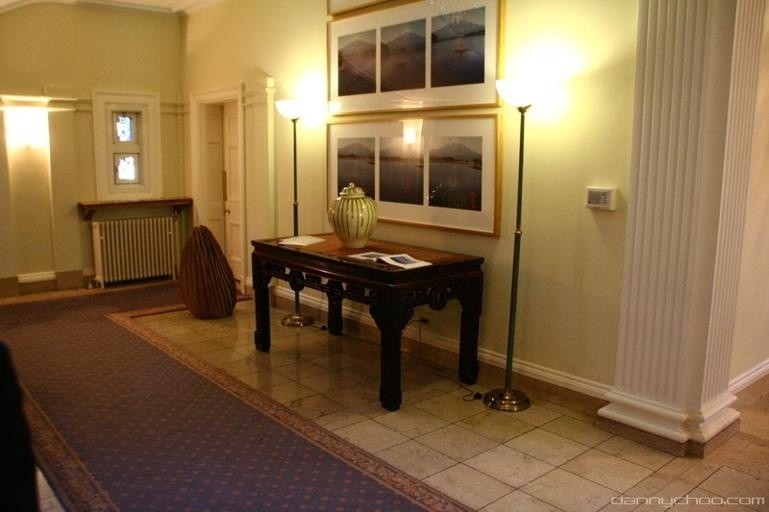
[343,251,434,270]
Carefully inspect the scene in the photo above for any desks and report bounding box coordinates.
[249,235,486,412]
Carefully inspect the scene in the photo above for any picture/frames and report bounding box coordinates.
[326,0,504,237]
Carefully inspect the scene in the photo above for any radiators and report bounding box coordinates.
[90,215,181,288]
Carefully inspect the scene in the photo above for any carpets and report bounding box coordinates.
[0,281,475,512]
